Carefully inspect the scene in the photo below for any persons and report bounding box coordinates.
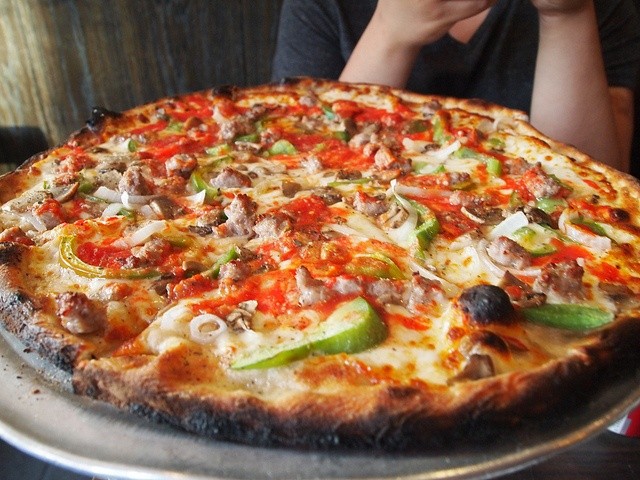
[270,0,640,173]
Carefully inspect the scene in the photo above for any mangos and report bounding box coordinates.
[1,75,639,454]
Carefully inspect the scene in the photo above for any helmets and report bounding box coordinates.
[0,334,639,479]
